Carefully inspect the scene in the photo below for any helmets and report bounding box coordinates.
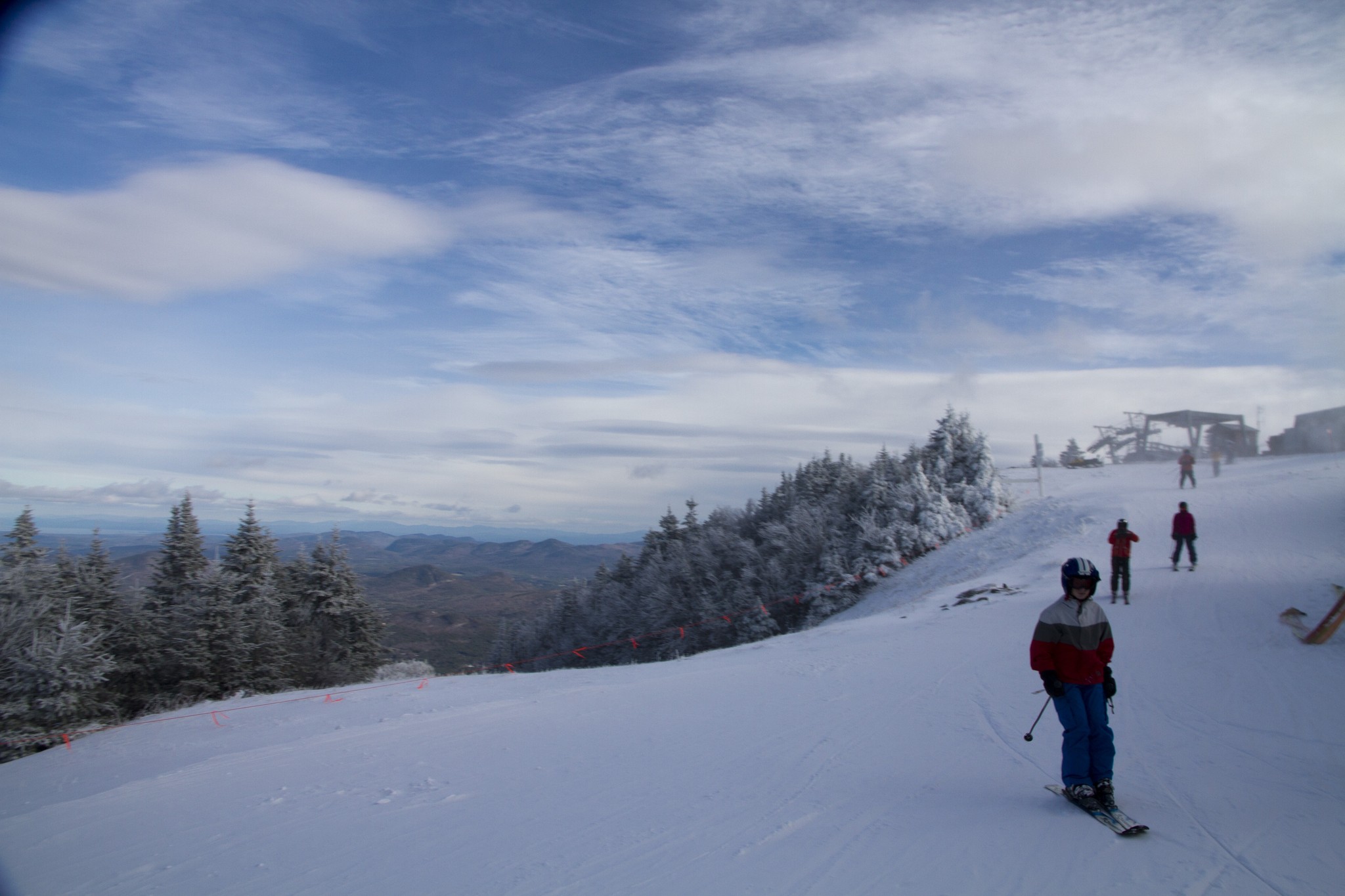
[1118,519,1127,528]
[1062,558,1101,598]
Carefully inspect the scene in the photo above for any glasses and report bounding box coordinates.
[1063,572,1098,589]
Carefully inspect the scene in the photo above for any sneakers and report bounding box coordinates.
[1095,778,1118,809]
[1065,784,1099,811]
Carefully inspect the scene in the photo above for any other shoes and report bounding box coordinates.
[1173,563,1178,571]
[1112,596,1116,603]
[1190,564,1197,570]
[1124,595,1129,604]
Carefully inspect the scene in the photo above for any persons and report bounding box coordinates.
[1108,519,1139,595]
[1171,502,1197,565]
[1179,449,1196,486]
[1030,558,1115,811]
[1210,448,1220,477]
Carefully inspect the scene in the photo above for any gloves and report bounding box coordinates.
[1103,666,1116,697]
[1172,533,1178,540]
[1040,669,1063,697]
[1190,533,1197,542]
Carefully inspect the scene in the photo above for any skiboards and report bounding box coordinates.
[1110,593,1131,605]
[1046,783,1148,835]
[1172,563,1197,572]
